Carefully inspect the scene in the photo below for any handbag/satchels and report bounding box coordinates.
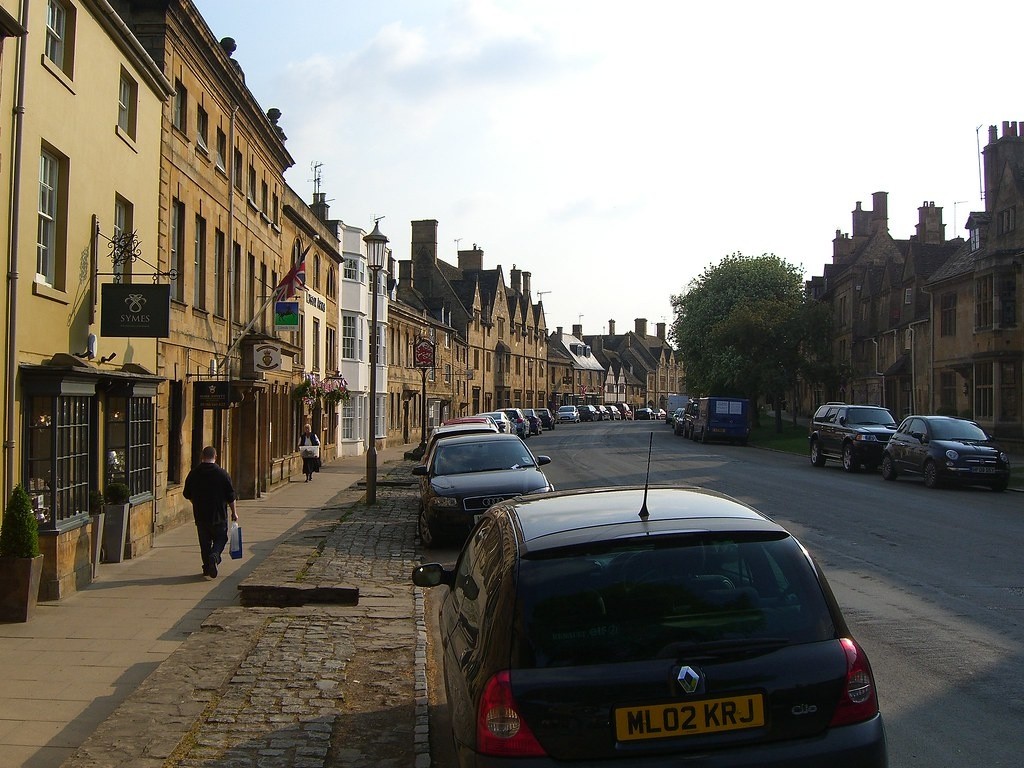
[229,522,242,559]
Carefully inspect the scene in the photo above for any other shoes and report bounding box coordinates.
[305,480,308,482]
[203,555,221,578]
[309,478,312,481]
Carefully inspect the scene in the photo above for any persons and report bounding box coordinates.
[183,446,238,579]
[297,423,321,482]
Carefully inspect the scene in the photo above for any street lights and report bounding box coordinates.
[360,218,391,510]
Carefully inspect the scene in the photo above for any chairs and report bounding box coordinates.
[530,550,761,626]
[442,446,507,474]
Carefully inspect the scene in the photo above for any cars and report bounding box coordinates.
[410,432,556,549]
[408,481,891,768]
[417,394,751,464]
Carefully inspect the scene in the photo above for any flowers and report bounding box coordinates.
[294,378,352,407]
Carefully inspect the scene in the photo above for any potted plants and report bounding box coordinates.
[102,482,132,562]
[89,491,106,577]
[0,482,45,621]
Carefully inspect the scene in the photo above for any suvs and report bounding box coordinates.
[878,415,1010,493]
[807,401,900,474]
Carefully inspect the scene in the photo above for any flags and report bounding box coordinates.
[275,255,307,301]
